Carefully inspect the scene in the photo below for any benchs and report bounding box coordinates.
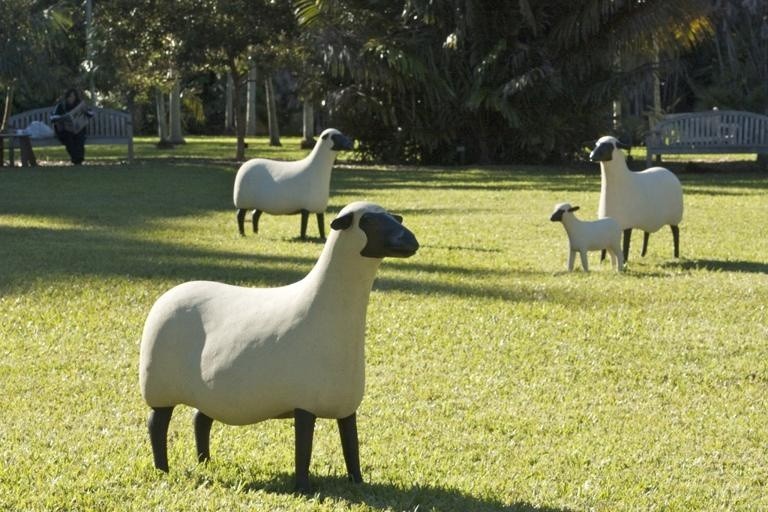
[7,104,136,164]
[647,107,768,174]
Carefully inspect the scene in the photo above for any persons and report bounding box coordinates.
[49,87,95,167]
